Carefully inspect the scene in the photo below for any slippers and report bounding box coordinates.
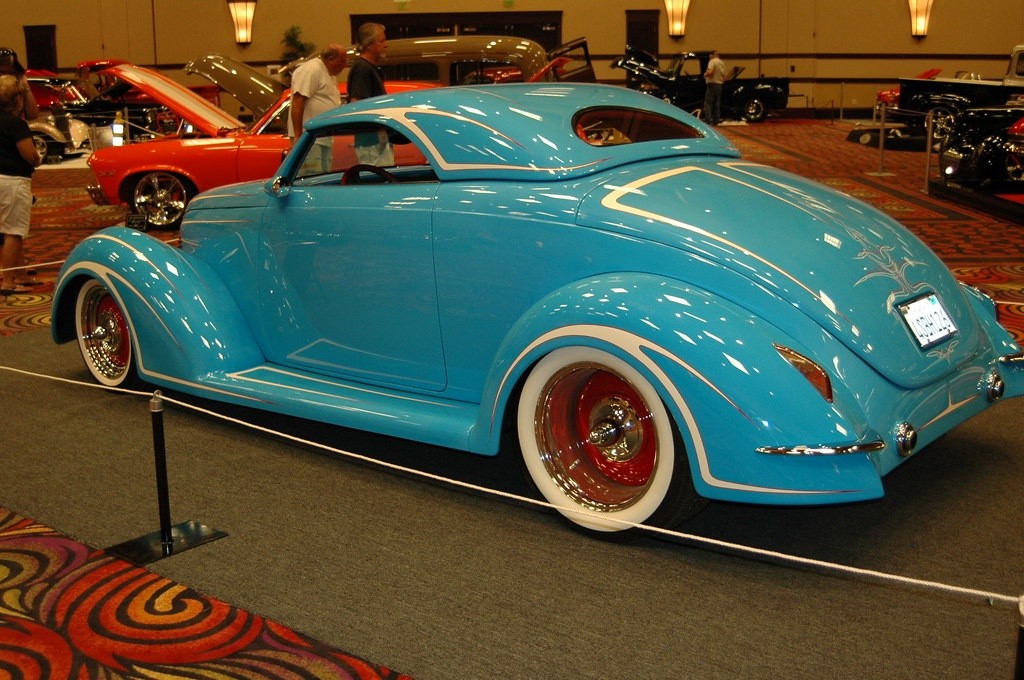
[0,284,30,295]
[15,278,43,286]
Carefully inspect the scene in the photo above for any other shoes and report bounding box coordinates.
[712,121,718,126]
[703,119,710,124]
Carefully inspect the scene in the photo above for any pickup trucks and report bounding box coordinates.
[893,45,1024,155]
[610,45,790,124]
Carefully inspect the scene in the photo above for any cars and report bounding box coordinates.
[48,83,1024,547]
[938,95,1024,195]
[23,36,597,232]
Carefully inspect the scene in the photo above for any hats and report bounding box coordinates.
[709,50,718,55]
[0,75,30,95]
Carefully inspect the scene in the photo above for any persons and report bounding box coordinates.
[346,24,395,167]
[703,50,725,126]
[0,46,44,293]
[288,41,346,178]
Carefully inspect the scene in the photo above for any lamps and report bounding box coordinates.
[664,0,691,42]
[907,0,934,42]
[226,0,257,45]
